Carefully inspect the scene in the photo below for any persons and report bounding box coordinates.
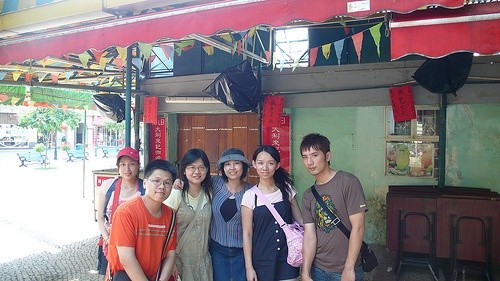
[96,146,146,276]
[172,148,255,281]
[105,159,177,281]
[240,145,302,281]
[300,133,368,281]
[163,148,213,281]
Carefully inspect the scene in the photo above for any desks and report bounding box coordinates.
[386,186,500,281]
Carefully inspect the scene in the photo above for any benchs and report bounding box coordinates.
[101,146,119,158]
[17,150,50,167]
[66,149,89,163]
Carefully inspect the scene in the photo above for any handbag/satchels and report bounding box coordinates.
[360,242,379,273]
[252,185,303,267]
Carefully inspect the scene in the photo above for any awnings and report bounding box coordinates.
[0,0,500,65]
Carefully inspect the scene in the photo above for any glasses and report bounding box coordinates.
[187,166,207,172]
[147,179,172,187]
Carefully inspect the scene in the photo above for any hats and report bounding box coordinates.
[217,147,250,171]
[116,146,139,162]
